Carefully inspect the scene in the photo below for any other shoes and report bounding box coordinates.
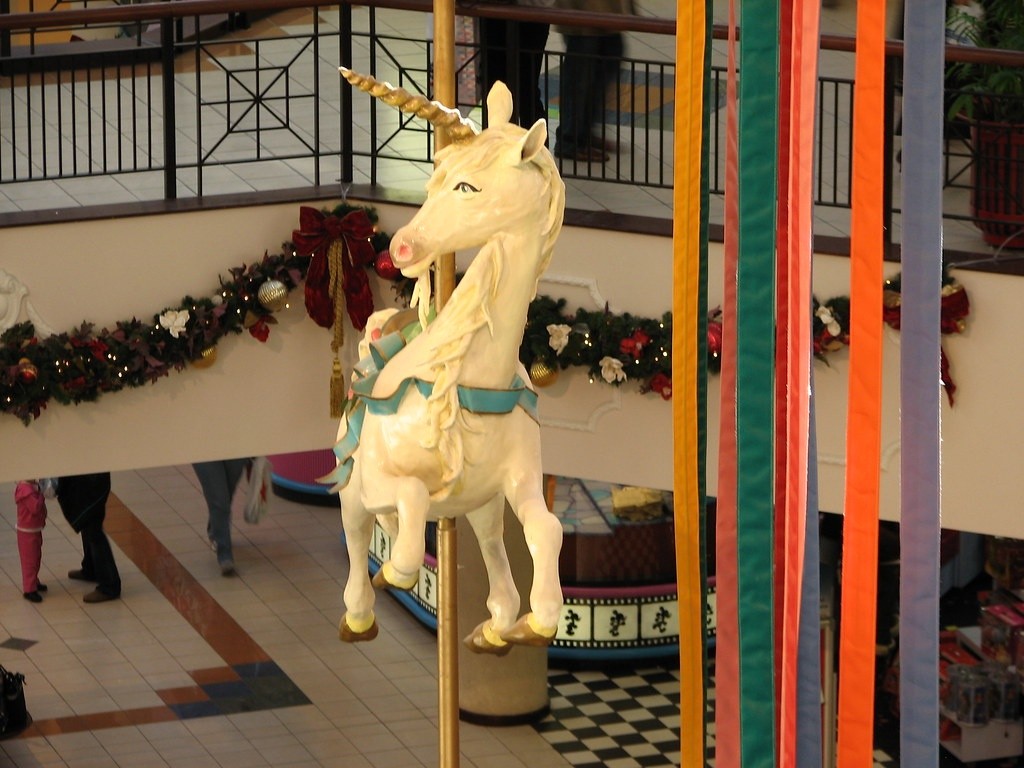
[553,141,609,163]
[218,556,235,576]
[37,584,47,591]
[24,591,42,603]
[68,569,94,581]
[83,588,120,603]
[209,535,217,553]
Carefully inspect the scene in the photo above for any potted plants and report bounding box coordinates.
[902,0,1024,249]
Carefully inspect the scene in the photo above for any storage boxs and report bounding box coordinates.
[981,604,1024,666]
[891,697,900,718]
[1011,629,1024,683]
[985,536,1024,590]
[940,641,980,668]
[939,659,953,702]
[938,718,960,740]
[885,667,899,695]
[996,587,1024,617]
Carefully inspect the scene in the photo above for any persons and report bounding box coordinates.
[14,480,48,602]
[54,472,121,603]
[192,459,245,576]
[477,0,636,163]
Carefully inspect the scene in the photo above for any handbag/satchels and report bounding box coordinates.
[0,662,26,741]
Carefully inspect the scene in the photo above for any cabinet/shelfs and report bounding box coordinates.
[940,535,1024,763]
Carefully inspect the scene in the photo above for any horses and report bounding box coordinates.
[312,65,565,658]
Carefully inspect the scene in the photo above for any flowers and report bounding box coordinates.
[0,195,969,437]
